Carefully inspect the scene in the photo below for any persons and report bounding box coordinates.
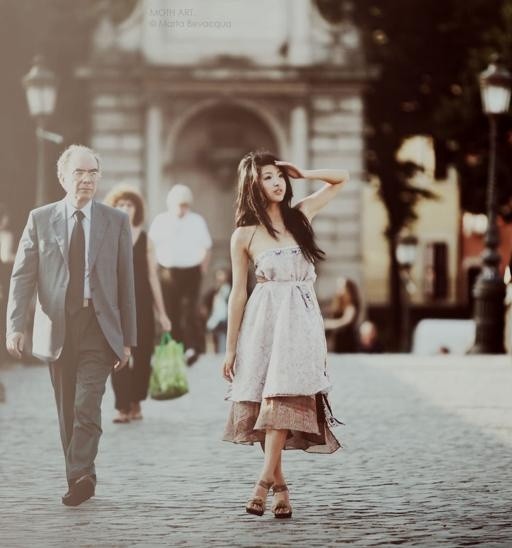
[219,151,350,520]
[152,185,233,367]
[6,144,139,508]
[102,183,174,423]
[322,276,364,353]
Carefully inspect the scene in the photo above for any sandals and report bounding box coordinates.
[273,484,294,519]
[247,482,265,516]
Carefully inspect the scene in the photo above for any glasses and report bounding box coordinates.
[72,169,100,183]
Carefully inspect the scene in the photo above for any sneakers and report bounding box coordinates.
[114,403,140,422]
[61,476,95,507]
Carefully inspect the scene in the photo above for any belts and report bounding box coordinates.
[80,298,93,308]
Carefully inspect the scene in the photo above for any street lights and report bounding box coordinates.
[466,53,511,357]
[17,50,63,206]
[394,225,421,353]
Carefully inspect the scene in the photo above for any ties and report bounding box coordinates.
[65,211,84,317]
